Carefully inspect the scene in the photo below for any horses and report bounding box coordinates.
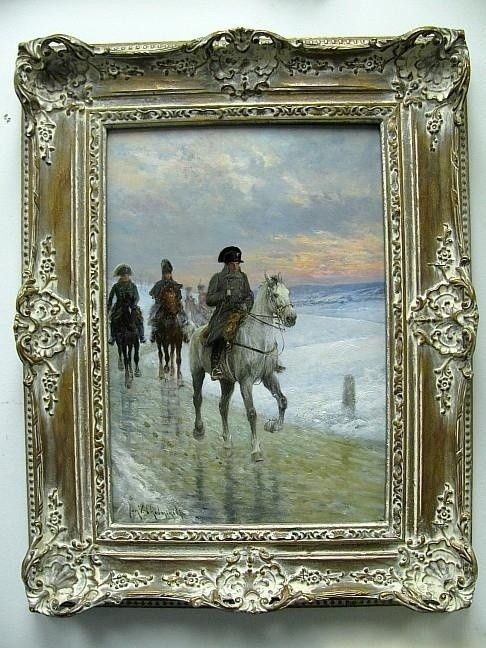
[188,269,298,463]
[107,294,143,389]
[154,283,186,389]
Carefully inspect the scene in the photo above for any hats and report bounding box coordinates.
[218,246,244,262]
[161,259,173,272]
[112,264,135,277]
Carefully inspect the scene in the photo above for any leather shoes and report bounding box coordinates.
[211,352,222,376]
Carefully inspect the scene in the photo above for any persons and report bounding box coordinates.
[184,289,197,312]
[204,261,288,381]
[107,273,146,344]
[148,271,190,343]
[198,288,207,312]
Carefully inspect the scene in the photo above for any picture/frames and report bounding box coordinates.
[14,27,479,619]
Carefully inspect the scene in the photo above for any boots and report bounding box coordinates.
[140,335,145,343]
[151,336,155,342]
[184,337,190,343]
[108,337,116,345]
